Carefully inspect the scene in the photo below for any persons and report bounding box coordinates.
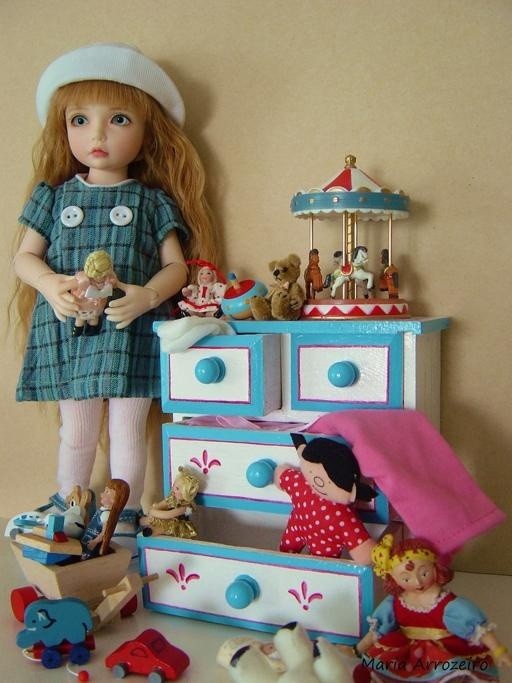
[5,39,511,682]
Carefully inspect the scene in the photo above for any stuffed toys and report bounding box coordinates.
[5,39,511,682]
